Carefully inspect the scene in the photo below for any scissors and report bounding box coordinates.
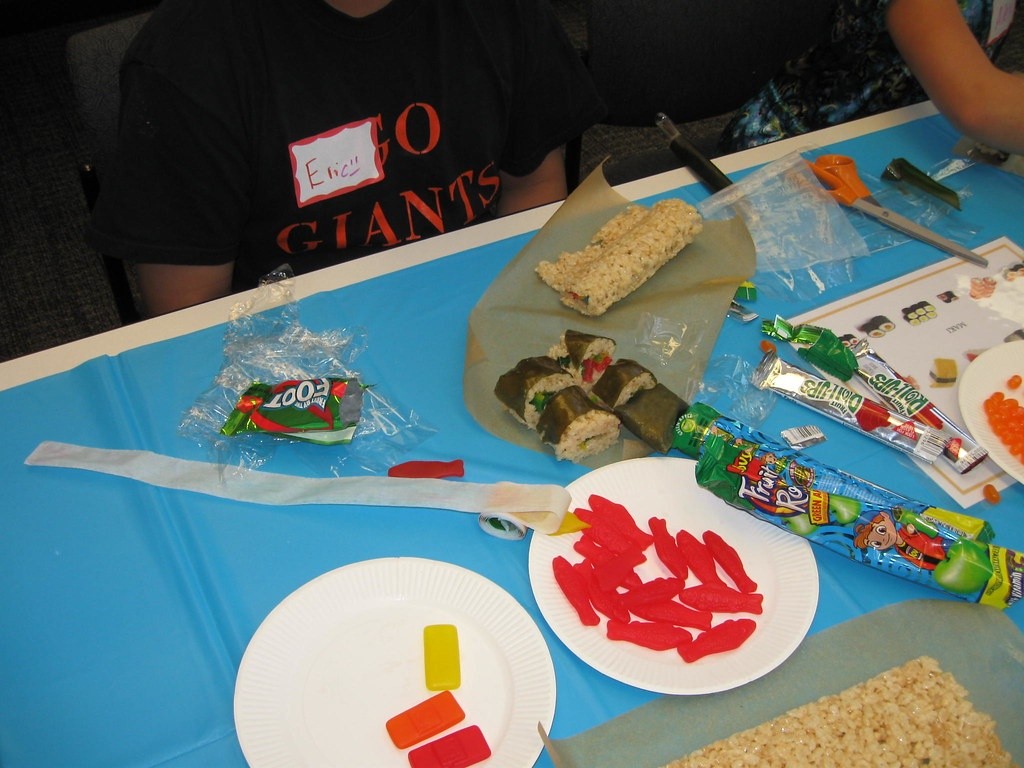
[788,155,989,266]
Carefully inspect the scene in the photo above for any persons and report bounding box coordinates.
[716,0,1024,156]
[85,0,607,320]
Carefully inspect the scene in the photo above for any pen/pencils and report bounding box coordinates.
[655,110,762,223]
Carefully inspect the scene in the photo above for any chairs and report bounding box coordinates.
[63,6,264,331]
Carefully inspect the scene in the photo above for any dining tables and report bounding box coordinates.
[0,100,1024,768]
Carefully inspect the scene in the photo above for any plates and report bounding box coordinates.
[233,556,557,768]
[958,340,1024,486]
[528,456,819,695]
